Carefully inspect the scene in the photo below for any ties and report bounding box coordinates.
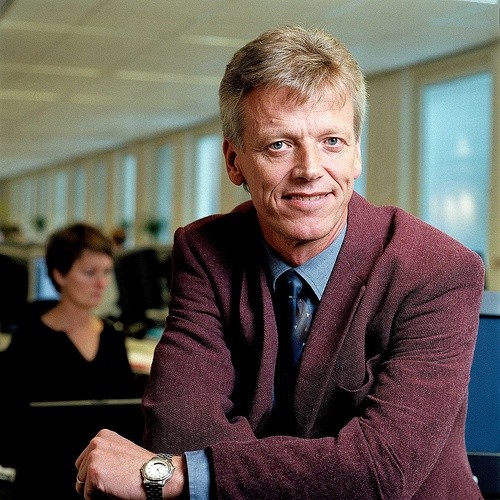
[272,269,318,435]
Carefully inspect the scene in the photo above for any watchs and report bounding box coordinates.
[140,452,176,500]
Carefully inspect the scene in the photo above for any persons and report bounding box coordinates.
[69,21,485,500]
[111,228,127,251]
[0,254,29,336]
[0,222,136,500]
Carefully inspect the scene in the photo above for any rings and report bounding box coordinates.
[77,477,85,484]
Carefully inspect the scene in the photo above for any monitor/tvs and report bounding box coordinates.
[28,249,63,303]
[463,289,499,457]
